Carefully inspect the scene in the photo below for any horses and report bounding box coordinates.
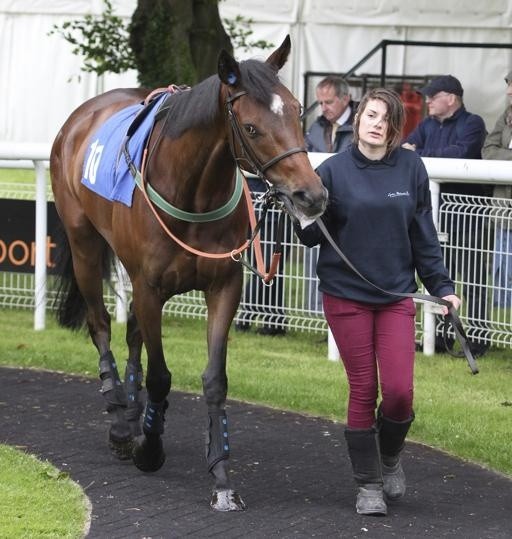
[47,33,332,516]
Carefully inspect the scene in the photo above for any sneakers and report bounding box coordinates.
[256,325,286,335]
[463,340,489,355]
[237,314,256,328]
[417,336,453,353]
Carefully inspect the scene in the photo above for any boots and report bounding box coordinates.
[344,425,388,517]
[378,403,415,499]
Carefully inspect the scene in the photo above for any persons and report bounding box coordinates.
[480,69,511,309]
[288,86,462,514]
[397,75,493,357]
[297,75,362,318]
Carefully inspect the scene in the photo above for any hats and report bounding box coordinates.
[415,75,463,96]
